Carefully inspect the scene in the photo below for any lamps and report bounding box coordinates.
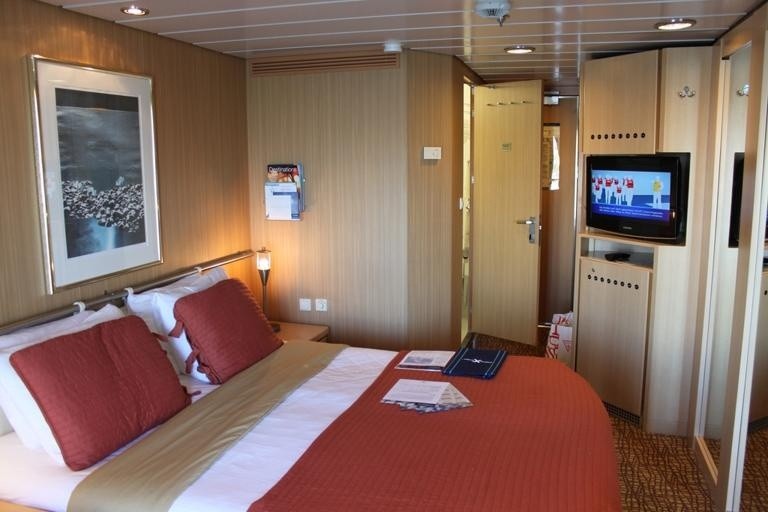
[255,246,272,316]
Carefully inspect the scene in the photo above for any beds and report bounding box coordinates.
[0,266,621,511]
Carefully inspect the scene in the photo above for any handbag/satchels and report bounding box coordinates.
[545,311,573,367]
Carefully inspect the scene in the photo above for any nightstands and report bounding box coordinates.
[273,321,331,345]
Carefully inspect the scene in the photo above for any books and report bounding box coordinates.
[379,379,473,415]
[393,347,456,373]
[444,344,507,379]
[263,162,304,221]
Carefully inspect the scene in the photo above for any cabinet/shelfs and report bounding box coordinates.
[703,46,753,437]
[571,41,711,437]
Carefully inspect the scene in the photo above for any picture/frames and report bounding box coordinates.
[24,51,165,297]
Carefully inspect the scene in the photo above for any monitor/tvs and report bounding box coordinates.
[728,151,768,249]
[585,151,691,245]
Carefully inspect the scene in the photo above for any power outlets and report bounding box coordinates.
[299,297,328,313]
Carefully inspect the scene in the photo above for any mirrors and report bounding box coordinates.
[692,2,766,512]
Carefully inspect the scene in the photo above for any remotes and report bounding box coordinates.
[604,251,631,261]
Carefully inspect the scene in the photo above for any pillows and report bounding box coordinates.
[156,267,230,384]
[1,314,192,471]
[174,278,282,383]
[4,303,142,466]
